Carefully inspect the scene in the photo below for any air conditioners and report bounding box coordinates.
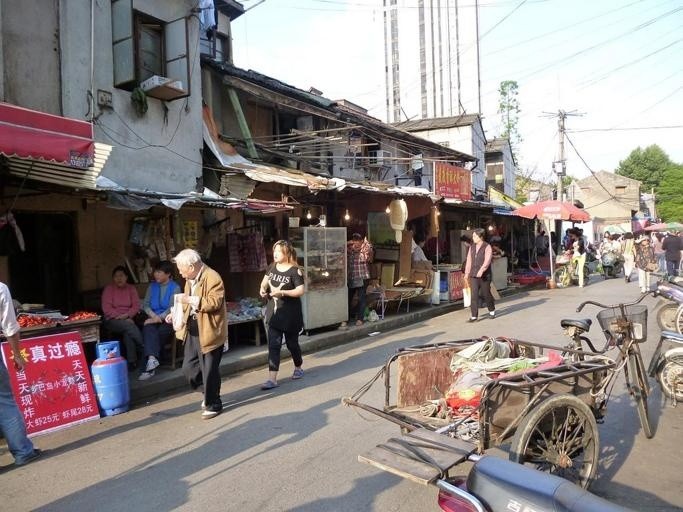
[367,150,393,168]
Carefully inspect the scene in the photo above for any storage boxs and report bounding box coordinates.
[140,74,185,100]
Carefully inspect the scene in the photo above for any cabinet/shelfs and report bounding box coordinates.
[289,227,349,336]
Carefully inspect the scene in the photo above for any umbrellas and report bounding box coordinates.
[510,199,591,281]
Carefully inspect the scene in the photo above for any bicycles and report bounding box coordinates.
[551,250,590,288]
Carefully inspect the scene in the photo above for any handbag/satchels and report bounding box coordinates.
[646,263,658,270]
[463,288,472,307]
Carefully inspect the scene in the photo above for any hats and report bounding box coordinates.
[669,230,677,236]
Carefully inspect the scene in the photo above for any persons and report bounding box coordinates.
[408,223,427,263]
[463,229,495,323]
[0,283,42,468]
[136,260,181,380]
[416,237,426,249]
[459,234,488,307]
[101,266,143,371]
[340,231,374,328]
[259,239,305,391]
[535,216,683,293]
[164,249,229,420]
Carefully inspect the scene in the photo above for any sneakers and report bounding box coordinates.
[24,449,41,464]
[201,400,223,419]
[356,321,362,326]
[489,311,496,318]
[146,359,160,371]
[138,371,155,381]
[466,318,477,322]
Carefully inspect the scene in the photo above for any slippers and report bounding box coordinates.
[292,369,304,379]
[261,380,278,389]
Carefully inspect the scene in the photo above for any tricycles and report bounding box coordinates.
[337,282,659,494]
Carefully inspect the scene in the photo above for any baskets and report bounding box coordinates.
[597,306,648,346]
[556,255,569,264]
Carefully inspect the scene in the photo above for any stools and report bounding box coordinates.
[142,325,185,369]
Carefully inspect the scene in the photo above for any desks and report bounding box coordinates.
[435,264,465,303]
[367,284,425,320]
[1,310,102,345]
[227,303,263,348]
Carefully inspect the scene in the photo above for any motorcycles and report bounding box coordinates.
[647,269,683,405]
[600,247,625,281]
[431,454,633,512]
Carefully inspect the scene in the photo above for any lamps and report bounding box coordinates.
[385,198,390,214]
[306,206,311,219]
[345,206,350,220]
[318,205,327,227]
